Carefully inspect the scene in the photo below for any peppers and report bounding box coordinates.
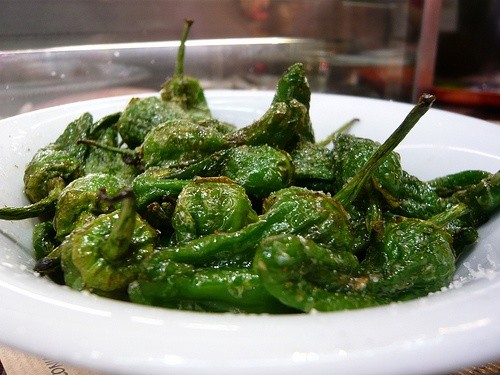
[0,16,500,312]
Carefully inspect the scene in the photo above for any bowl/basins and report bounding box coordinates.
[0,83,499,372]
